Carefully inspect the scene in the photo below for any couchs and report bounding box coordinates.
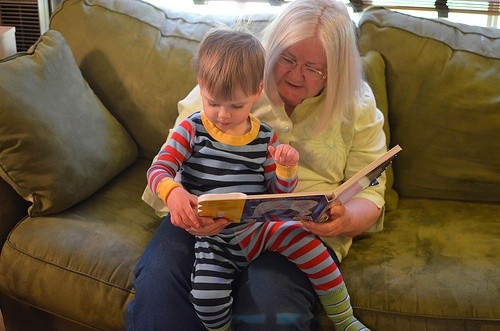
[0,1,500,330]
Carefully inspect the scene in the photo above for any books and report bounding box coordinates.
[197,144,402,224]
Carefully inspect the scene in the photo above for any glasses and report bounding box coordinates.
[276,53,329,81]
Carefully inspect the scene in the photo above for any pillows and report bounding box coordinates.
[358,51,399,209]
[0,30,139,218]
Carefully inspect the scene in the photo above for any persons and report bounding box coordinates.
[147,21,372,331]
[124,0,388,331]
